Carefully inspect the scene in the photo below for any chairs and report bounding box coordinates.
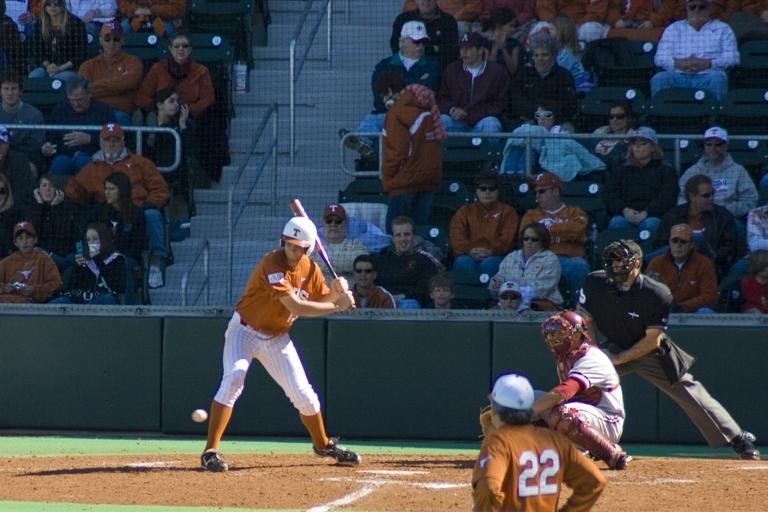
[336,15,767,313]
[1,0,270,305]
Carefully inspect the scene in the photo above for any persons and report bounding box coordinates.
[577,240,761,461]
[200,217,361,472]
[533,309,632,469]
[471,374,606,512]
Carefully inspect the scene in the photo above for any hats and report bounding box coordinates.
[531,172,562,188]
[668,222,693,240]
[686,0,712,6]
[101,22,124,38]
[704,126,728,144]
[280,215,318,256]
[497,281,522,298]
[323,203,347,221]
[13,221,36,239]
[399,21,432,44]
[628,126,658,145]
[454,31,484,48]
[486,373,535,411]
[100,122,124,139]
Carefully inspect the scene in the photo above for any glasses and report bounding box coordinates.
[476,185,498,192]
[354,268,375,274]
[102,36,122,42]
[695,187,715,199]
[324,219,345,225]
[500,295,521,300]
[522,235,542,242]
[532,187,554,195]
[534,110,554,120]
[704,141,726,147]
[669,237,691,244]
[170,42,192,49]
[608,113,629,120]
[688,4,709,11]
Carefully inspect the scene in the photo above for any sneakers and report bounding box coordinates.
[605,441,630,470]
[312,437,362,466]
[200,451,229,473]
[731,439,761,461]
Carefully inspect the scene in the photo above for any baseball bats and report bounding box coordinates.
[290,197,355,312]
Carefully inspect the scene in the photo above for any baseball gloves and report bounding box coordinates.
[479,404,497,438]
